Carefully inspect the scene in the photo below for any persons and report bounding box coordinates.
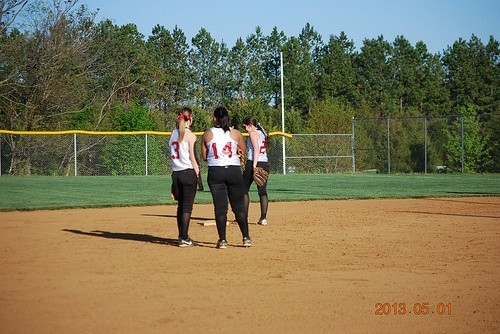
[201,107,254,249]
[232,116,269,225]
[169,107,203,247]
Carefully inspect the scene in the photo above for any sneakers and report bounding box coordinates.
[243,236,254,247]
[216,239,228,249]
[258,217,268,226]
[178,238,197,248]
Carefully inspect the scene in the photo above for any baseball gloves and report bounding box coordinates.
[253,167,268,186]
